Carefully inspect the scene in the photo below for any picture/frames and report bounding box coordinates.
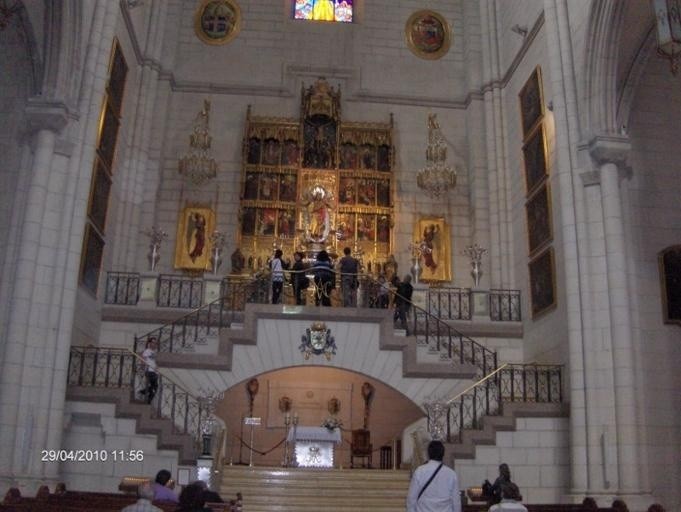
[73,35,130,300]
[516,63,559,321]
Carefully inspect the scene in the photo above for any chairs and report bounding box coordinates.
[350,429,373,468]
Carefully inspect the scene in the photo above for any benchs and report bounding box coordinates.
[461,494,666,512]
[1,480,244,512]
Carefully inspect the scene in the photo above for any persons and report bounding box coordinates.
[189,213,206,264]
[582,497,598,512]
[337,247,362,307]
[485,463,528,512]
[4,482,65,504]
[647,503,666,512]
[423,224,440,274]
[301,192,336,240]
[313,251,335,306]
[407,440,462,512]
[390,271,414,336]
[138,337,160,404]
[376,275,390,308]
[269,249,291,304]
[305,120,334,154]
[121,470,224,512]
[611,500,629,512]
[289,252,305,304]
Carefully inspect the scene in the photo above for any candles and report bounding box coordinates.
[286,411,298,417]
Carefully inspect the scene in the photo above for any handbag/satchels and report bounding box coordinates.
[301,278,310,289]
[352,279,359,290]
